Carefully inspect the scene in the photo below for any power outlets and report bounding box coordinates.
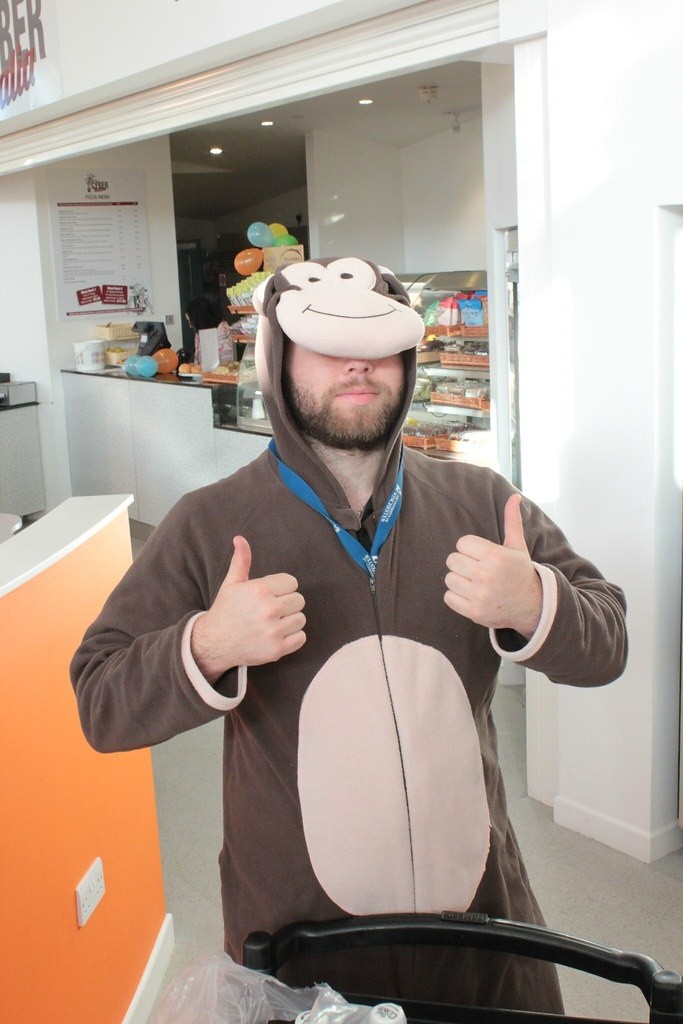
[76,857,106,928]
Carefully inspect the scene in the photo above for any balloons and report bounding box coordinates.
[153,348,179,373]
[247,222,274,247]
[269,223,299,246]
[125,354,158,378]
[234,248,263,275]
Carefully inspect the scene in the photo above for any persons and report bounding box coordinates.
[185,297,234,367]
[69,256,628,1015]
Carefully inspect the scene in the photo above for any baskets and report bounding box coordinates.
[439,352,489,366]
[431,391,490,410]
[74,340,105,372]
[401,427,468,452]
[459,325,488,337]
[424,324,460,338]
[105,349,137,364]
[96,323,140,341]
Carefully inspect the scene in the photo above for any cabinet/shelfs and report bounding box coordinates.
[237,272,492,460]
